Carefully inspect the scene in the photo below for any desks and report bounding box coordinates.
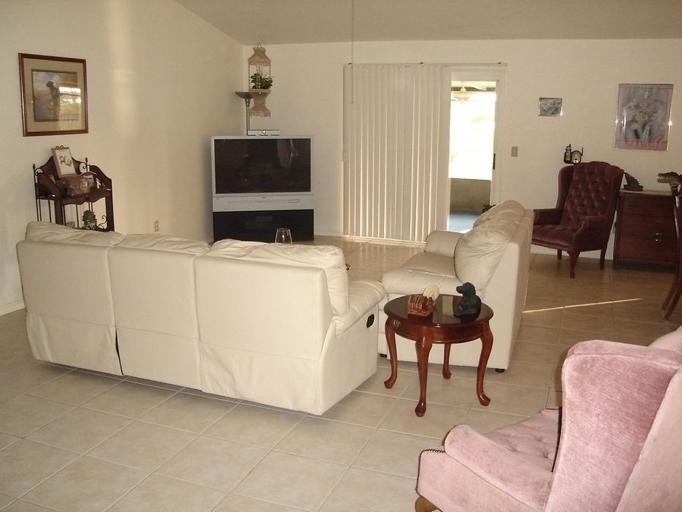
[381,293,496,419]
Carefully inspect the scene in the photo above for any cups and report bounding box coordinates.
[275,227,294,252]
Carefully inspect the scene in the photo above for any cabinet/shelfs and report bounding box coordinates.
[612,187,677,269]
[32,154,115,234]
[210,208,316,245]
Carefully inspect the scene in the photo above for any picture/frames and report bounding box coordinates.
[538,96,564,120]
[50,145,77,180]
[15,51,89,137]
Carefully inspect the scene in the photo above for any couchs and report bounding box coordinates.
[532,160,626,280]
[416,327,682,511]
[376,198,538,376]
[15,220,390,418]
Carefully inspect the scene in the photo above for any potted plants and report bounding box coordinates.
[245,45,274,117]
[81,209,97,230]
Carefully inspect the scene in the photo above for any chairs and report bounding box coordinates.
[659,191,682,321]
